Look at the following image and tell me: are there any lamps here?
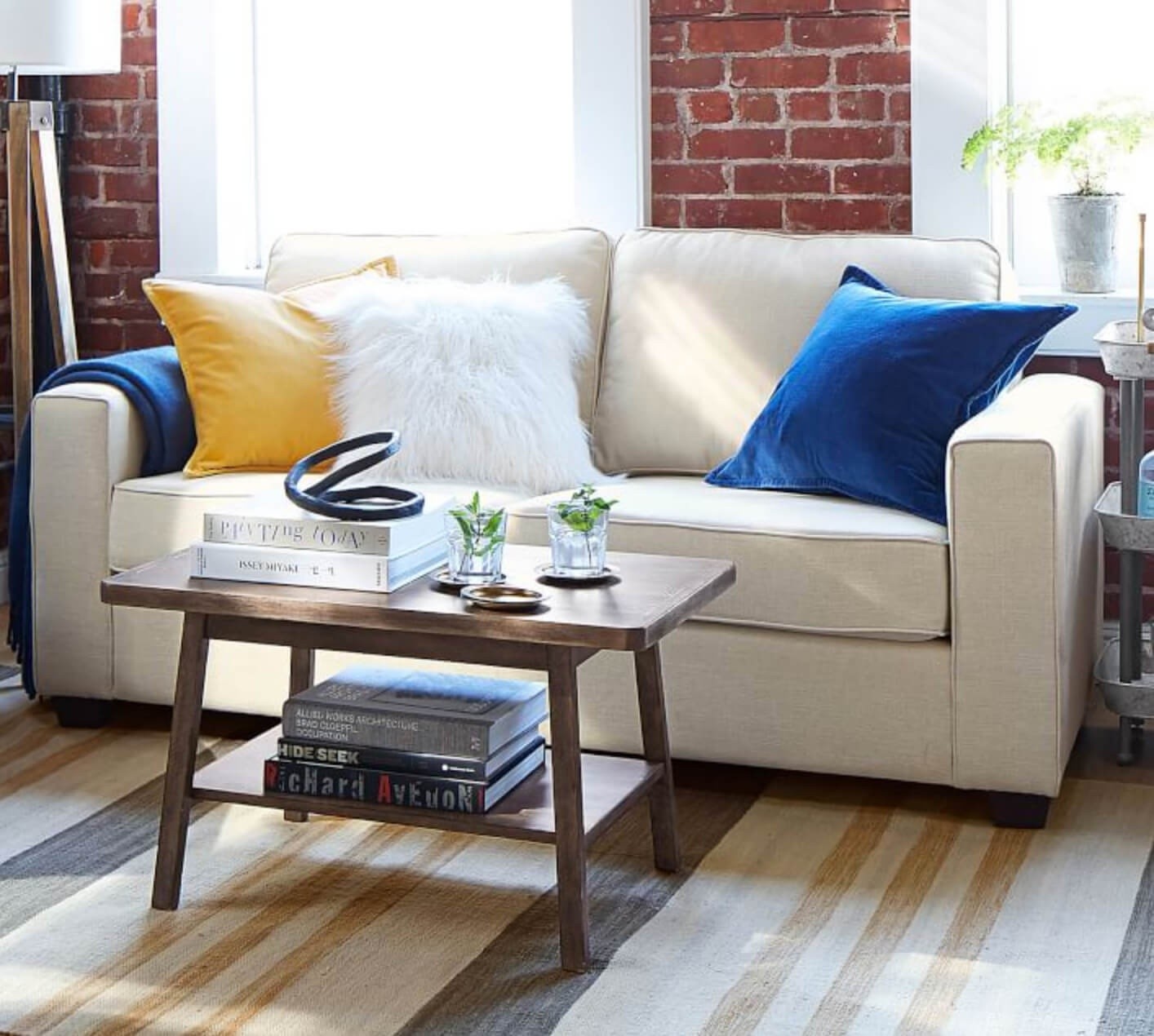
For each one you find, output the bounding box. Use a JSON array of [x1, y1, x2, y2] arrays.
[[0, 0, 121, 465]]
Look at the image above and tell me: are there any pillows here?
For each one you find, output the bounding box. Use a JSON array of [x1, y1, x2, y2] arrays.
[[704, 264, 1078, 525], [138, 257, 397, 479], [346, 272, 607, 490]]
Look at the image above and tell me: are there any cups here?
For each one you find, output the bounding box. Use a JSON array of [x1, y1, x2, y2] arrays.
[[546, 502, 610, 575], [443, 512, 508, 583]]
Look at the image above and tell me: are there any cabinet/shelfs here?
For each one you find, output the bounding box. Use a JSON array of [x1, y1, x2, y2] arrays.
[[1094, 318, 1154, 766]]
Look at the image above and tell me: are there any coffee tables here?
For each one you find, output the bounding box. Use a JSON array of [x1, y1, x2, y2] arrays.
[[102, 536, 742, 975]]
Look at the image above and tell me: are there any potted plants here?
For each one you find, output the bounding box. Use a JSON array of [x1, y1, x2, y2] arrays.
[[537, 477, 622, 579], [961, 105, 1144, 294], [439, 492, 509, 585]]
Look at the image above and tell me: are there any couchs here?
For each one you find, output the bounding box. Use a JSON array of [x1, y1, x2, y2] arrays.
[[23, 226, 1106, 831]]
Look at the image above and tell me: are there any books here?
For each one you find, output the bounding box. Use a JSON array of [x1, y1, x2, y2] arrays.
[[188, 535, 453, 594], [204, 499, 454, 556], [264, 735, 546, 815], [277, 724, 539, 782], [283, 667, 549, 759]]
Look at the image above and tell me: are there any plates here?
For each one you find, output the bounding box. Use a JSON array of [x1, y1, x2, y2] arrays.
[[533, 563, 614, 581], [427, 567, 509, 585], [461, 584, 550, 610]]
